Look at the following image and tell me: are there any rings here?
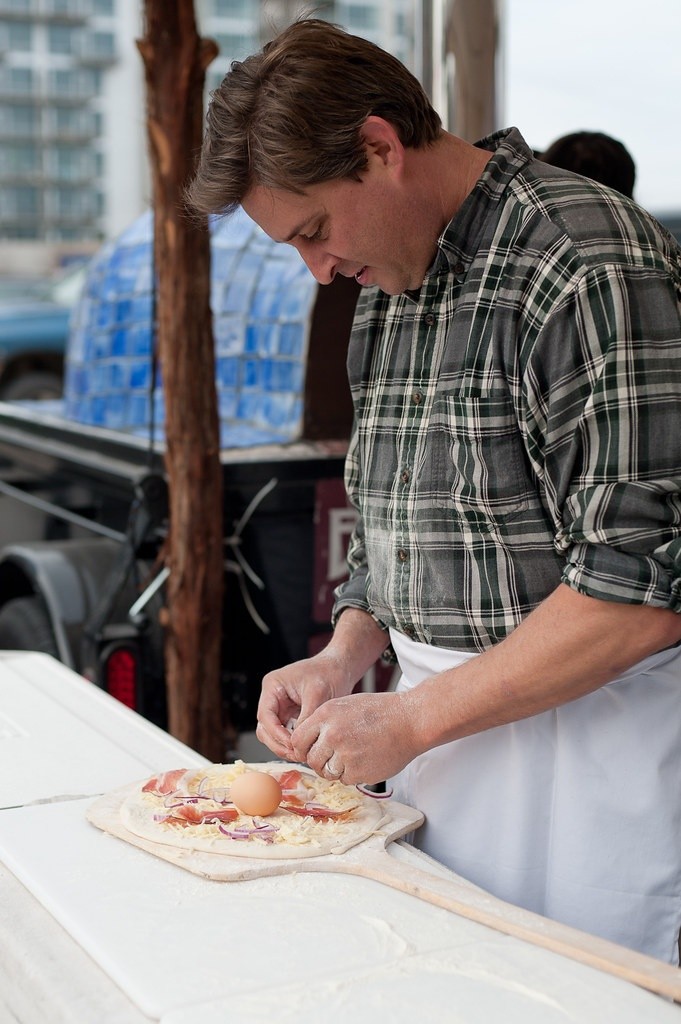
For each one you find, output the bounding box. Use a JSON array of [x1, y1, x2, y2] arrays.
[[325, 762, 344, 775]]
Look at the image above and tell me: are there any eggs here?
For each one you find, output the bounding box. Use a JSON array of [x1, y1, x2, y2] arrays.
[[230, 771, 283, 817]]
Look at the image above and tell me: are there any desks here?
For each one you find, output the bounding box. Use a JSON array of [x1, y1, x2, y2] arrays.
[[1, 398, 351, 672], [0, 647, 681, 1024]]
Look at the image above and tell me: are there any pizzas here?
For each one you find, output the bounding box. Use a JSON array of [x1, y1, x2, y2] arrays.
[[117, 759, 390, 859]]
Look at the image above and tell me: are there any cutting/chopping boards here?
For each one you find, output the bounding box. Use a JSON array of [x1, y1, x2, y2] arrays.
[[89, 763, 681, 1004]]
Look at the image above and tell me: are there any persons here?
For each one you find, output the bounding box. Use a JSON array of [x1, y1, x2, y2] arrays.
[[544, 132, 636, 200], [180, 17, 681, 962]]
